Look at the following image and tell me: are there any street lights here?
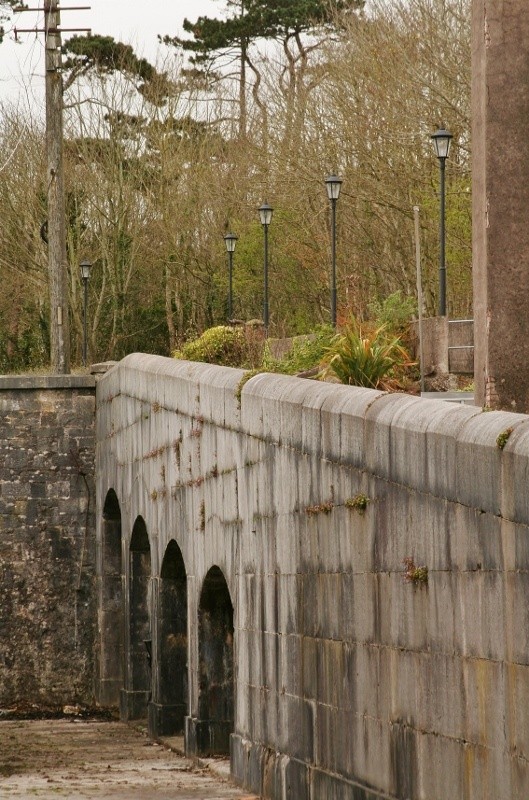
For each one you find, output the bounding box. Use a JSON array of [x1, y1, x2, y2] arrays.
[[325, 175, 344, 327], [257, 204, 274, 323], [223, 233, 239, 318], [429, 128, 455, 318], [80, 260, 92, 360]]
